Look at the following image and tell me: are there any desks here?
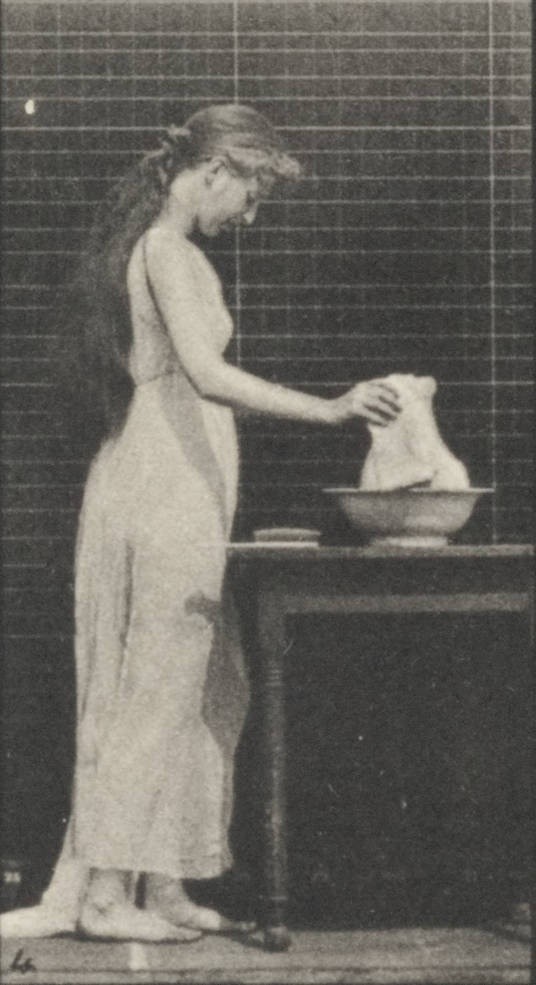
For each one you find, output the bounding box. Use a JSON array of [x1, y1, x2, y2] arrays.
[[228, 541, 535, 955]]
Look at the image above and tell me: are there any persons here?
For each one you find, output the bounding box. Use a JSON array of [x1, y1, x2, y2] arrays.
[[72, 103, 403, 946]]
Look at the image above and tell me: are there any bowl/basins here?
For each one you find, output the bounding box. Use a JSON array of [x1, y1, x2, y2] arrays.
[[320, 487, 496, 546]]
